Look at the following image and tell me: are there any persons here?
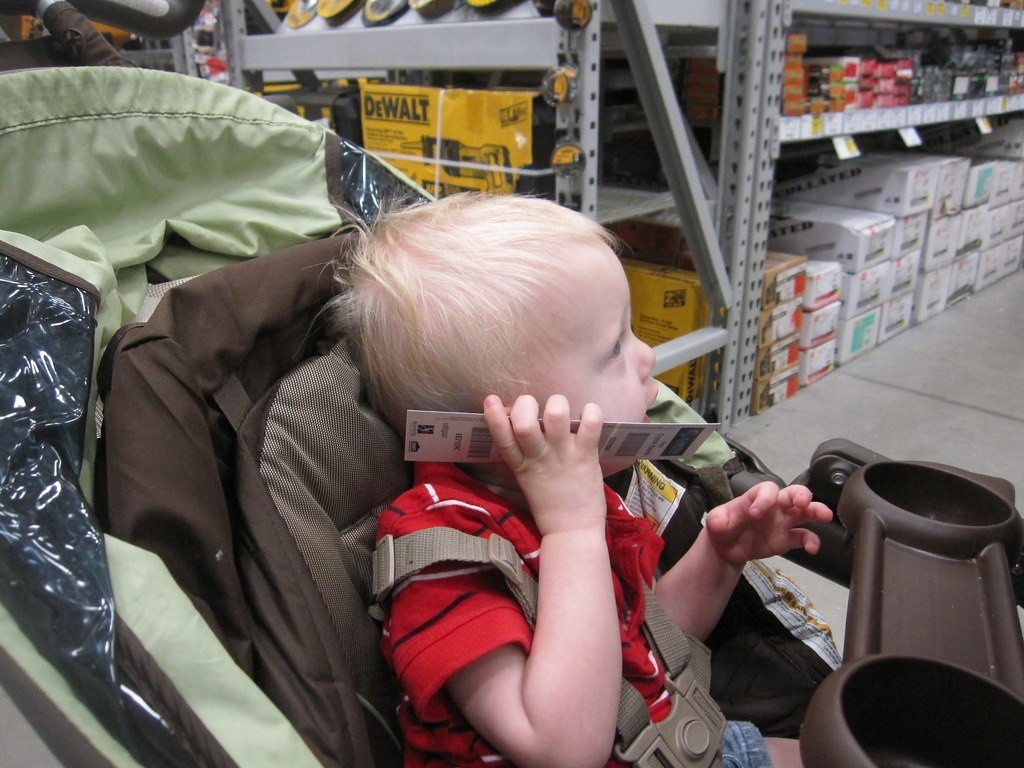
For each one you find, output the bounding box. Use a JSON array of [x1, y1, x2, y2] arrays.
[[337, 189, 834, 768]]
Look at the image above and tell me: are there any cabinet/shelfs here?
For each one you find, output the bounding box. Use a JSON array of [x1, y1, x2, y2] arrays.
[[224, 0, 770, 427], [736, 0, 1024, 419]]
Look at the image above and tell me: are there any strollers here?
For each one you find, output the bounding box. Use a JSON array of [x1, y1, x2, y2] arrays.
[[0, 0, 1024, 768]]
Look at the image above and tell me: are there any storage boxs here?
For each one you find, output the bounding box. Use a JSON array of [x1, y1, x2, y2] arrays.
[[254, 0, 1024, 415]]
